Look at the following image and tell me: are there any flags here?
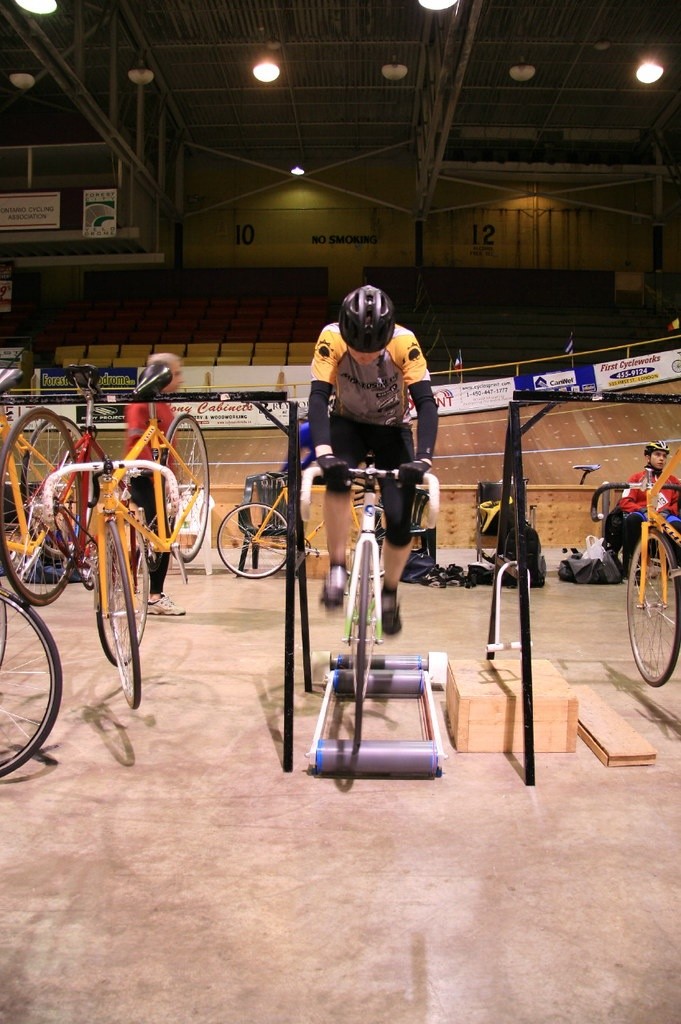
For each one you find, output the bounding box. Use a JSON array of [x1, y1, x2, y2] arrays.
[[564, 334, 575, 354], [667, 318, 680, 332], [454, 353, 460, 369]]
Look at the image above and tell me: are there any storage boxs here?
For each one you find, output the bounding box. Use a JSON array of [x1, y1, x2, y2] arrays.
[[445, 657, 578, 751]]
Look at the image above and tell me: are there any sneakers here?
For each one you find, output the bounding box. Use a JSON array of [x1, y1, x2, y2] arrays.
[[146, 593, 186, 615], [445, 563, 466, 587], [419, 564, 446, 588]]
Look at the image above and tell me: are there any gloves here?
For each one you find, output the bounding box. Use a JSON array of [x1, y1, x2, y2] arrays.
[[317, 454, 349, 484], [659, 510, 671, 518], [398, 459, 431, 485], [640, 507, 648, 518]]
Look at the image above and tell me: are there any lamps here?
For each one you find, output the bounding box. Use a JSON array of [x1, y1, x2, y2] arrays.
[[381, 55, 408, 81], [8, 70, 36, 88], [508, 55, 536, 82], [253, 54, 280, 82], [127, 50, 155, 86]]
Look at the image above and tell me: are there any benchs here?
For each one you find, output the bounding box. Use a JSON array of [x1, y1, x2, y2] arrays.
[[366, 294, 681, 389]]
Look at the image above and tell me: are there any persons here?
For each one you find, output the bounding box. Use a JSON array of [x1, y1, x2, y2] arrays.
[[124, 351, 186, 615], [308, 283, 440, 636], [617, 437, 680, 584]]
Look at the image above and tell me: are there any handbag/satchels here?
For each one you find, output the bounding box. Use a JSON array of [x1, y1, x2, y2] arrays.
[[559, 550, 625, 584], [581, 535, 607, 561], [465, 562, 494, 588], [400, 552, 435, 583]]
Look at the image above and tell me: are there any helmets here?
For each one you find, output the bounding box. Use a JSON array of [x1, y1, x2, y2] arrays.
[[644, 440, 670, 456], [339, 285, 395, 353]]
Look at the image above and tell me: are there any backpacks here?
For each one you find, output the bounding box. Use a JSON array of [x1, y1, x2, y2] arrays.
[[504, 525, 546, 588]]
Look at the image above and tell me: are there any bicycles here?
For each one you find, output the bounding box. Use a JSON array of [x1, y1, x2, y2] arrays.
[[216, 471, 387, 580], [590, 482, 681, 687], [299, 467, 439, 754], [0, 582, 64, 777], [42, 365, 211, 709], [0, 363, 149, 666]]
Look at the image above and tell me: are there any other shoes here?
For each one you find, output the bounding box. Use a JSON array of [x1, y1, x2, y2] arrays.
[[323, 564, 346, 607], [381, 589, 401, 635]]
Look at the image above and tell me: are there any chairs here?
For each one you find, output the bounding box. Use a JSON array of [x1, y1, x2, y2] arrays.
[[476, 481, 534, 558], [171, 489, 214, 576], [234, 471, 287, 575], [0, 294, 329, 381], [408, 488, 430, 557]]
